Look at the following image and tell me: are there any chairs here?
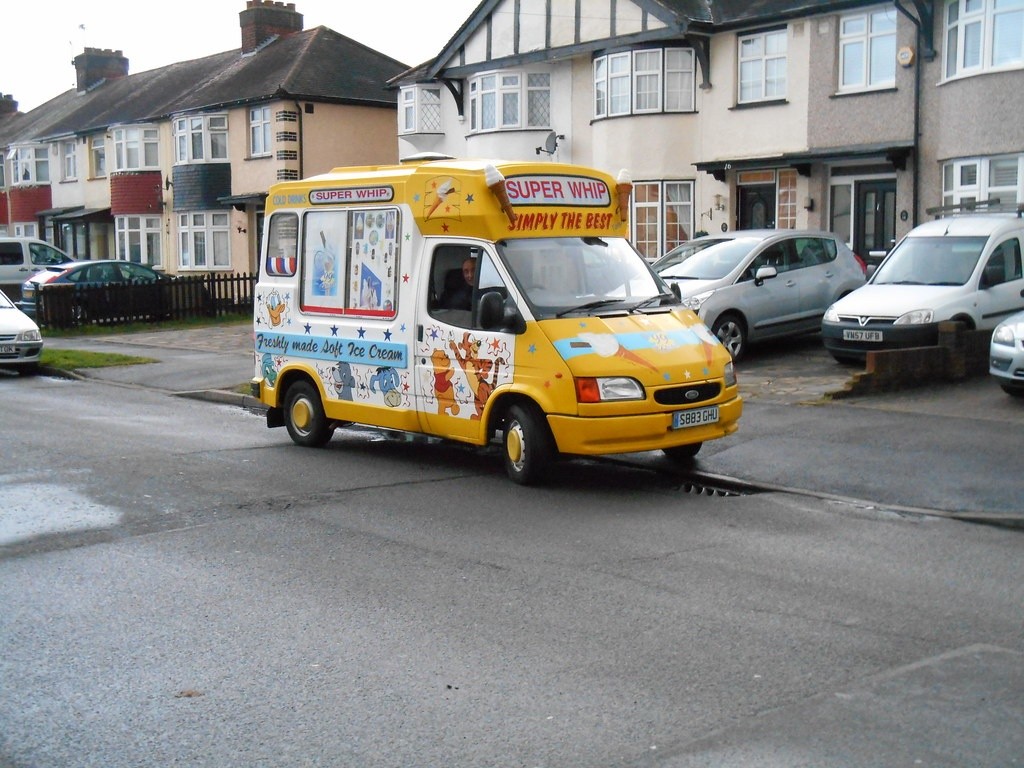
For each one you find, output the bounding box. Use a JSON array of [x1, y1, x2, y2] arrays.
[[760, 245, 783, 267], [442, 268, 473, 308], [90, 269, 104, 283]]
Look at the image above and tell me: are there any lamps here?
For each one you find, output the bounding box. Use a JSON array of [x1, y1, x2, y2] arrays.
[[236, 221, 247, 233], [803, 197, 814, 212], [713, 194, 724, 211]]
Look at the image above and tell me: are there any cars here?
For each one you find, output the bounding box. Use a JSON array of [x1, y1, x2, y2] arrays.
[[0, 289, 44, 376], [14, 258, 208, 328], [603, 227, 868, 366], [988, 289, 1024, 396], [820, 213, 1024, 384]]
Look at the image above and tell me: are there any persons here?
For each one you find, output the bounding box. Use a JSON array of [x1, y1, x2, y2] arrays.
[[444, 257, 477, 311]]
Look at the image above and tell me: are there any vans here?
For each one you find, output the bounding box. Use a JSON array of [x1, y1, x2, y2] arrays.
[[0, 238, 106, 309]]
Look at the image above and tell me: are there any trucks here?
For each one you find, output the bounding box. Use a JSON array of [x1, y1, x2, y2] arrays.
[[249, 158, 744, 487]]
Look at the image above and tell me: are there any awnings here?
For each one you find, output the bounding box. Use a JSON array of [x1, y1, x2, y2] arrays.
[[785, 142, 913, 176], [35, 205, 84, 246], [217, 192, 269, 212], [692, 156, 811, 182], [49, 208, 114, 260]]
